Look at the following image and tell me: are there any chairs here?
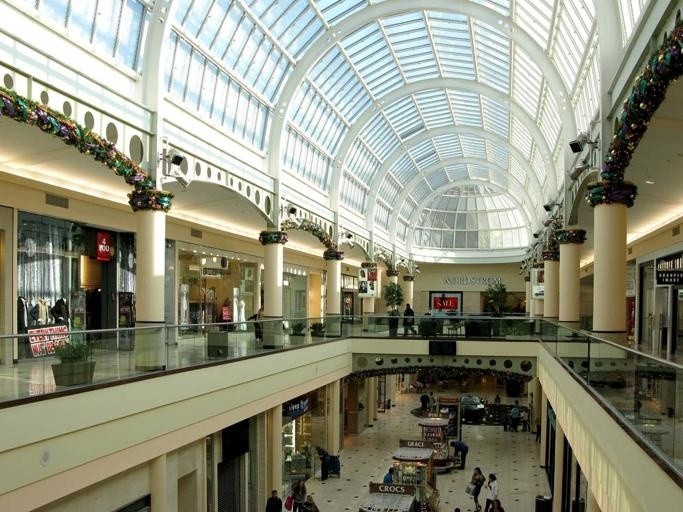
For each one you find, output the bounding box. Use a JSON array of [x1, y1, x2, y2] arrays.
[[421, 321, 436, 340]]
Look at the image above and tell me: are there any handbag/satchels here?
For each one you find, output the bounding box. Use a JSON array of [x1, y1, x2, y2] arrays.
[[284, 495, 294, 511], [464, 481, 476, 496]]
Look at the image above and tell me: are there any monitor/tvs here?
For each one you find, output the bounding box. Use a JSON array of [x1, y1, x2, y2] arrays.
[[429, 340, 456, 356], [220, 418, 249, 463]]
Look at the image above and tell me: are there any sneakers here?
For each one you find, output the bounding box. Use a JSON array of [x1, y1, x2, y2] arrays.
[[473, 505, 481, 512]]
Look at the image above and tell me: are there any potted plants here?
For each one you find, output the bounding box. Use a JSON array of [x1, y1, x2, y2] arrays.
[[384, 282, 404, 336], [289, 322, 305, 346], [488, 283, 507, 336], [52, 342, 97, 386]]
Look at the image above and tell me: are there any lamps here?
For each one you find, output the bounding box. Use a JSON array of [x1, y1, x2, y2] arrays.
[[342, 231, 352, 238], [570, 132, 595, 153], [283, 203, 296, 214], [534, 229, 543, 238], [544, 199, 561, 211], [160, 148, 183, 166]]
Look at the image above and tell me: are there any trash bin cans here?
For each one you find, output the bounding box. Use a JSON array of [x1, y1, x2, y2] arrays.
[[535, 495, 553, 512], [572, 497, 585, 512], [207, 330, 228, 361]]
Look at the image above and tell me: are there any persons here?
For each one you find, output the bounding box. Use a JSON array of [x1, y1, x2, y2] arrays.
[[289, 479, 306, 512], [264, 490, 282, 512], [453, 507, 460, 512], [400, 303, 417, 337], [358, 281, 367, 293], [49, 297, 71, 325], [427, 391, 435, 412], [469, 467, 486, 512], [419, 391, 429, 412], [246, 308, 263, 340], [484, 473, 498, 512], [314, 445, 330, 482], [30, 298, 53, 325], [414, 378, 424, 394], [534, 415, 540, 444], [488, 499, 505, 512], [493, 393, 500, 404], [502, 406, 511, 432], [449, 441, 468, 470], [382, 466, 394, 485], [17, 295, 28, 331], [509, 404, 519, 433], [519, 406, 527, 432], [218, 297, 231, 331], [300, 494, 319, 512]]
